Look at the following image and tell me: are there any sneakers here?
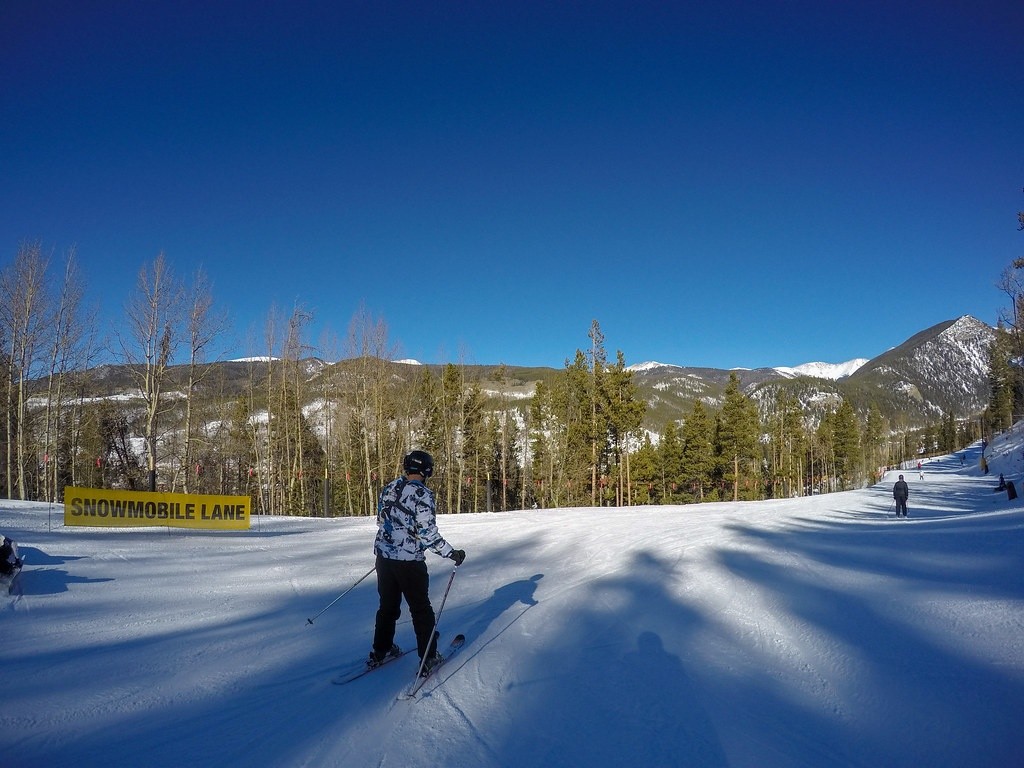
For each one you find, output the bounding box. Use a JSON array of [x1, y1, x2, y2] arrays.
[[370, 642, 399, 663], [419, 649, 443, 676]]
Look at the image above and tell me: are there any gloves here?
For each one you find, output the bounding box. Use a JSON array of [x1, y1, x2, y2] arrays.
[[14, 558, 23, 569], [447, 549, 466, 566]]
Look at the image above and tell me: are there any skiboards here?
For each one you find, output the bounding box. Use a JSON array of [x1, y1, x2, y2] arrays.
[[331, 630, 466, 700]]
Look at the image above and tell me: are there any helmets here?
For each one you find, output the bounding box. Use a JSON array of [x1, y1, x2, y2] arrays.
[[403, 451, 434, 478]]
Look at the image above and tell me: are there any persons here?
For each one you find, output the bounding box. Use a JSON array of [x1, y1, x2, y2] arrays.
[[918, 462, 921, 469], [369, 450, 466, 672], [893, 475, 908, 519], [0, 535, 21, 577], [919, 470, 924, 480]]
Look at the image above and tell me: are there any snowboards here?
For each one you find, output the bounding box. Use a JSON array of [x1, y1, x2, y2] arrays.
[[7, 555, 26, 597]]
